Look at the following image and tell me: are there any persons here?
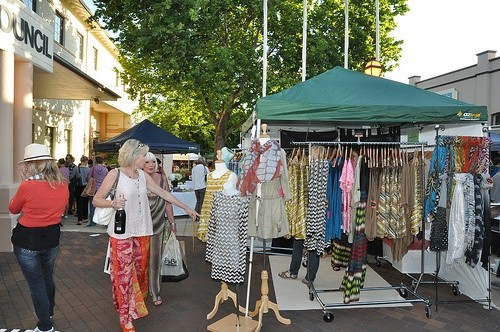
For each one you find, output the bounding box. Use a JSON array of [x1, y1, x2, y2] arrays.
[[279, 145, 326, 283], [196, 161, 238, 241], [154, 159, 209, 223], [205, 181, 249, 283], [91, 139, 200, 332], [489, 156, 500, 205], [56, 154, 112, 228], [141, 152, 177, 305], [9, 143, 70, 332]]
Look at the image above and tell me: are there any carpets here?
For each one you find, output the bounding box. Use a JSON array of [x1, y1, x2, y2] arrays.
[[269, 255, 413, 311]]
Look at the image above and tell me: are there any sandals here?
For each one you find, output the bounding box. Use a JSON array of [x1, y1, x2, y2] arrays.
[[278, 271, 297, 278], [302, 277, 313, 286], [152, 295, 163, 306], [331, 262, 340, 270], [120, 321, 136, 331]]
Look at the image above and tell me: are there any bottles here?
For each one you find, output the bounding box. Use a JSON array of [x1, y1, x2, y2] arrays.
[[114, 194, 126, 234]]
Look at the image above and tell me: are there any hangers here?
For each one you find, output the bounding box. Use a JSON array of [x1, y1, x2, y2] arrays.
[[290, 142, 420, 168]]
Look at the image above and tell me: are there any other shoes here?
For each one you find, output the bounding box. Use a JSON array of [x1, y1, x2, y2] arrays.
[[61, 213, 68, 218], [77, 218, 88, 224]]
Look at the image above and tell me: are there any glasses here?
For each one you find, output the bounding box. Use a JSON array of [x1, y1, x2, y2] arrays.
[[136, 140, 144, 150]]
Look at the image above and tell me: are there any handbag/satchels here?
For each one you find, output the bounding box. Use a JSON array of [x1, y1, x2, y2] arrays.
[[92, 167, 121, 225], [80, 166, 96, 197], [160, 229, 190, 282], [104, 240, 111, 274], [69, 165, 83, 186], [204, 165, 207, 182]]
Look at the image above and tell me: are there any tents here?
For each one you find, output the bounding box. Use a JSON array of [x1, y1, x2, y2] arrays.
[[242, 65, 491, 316], [94, 118, 201, 191]]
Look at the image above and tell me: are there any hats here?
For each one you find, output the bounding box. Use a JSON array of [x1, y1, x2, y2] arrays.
[[16, 143, 57, 164]]
